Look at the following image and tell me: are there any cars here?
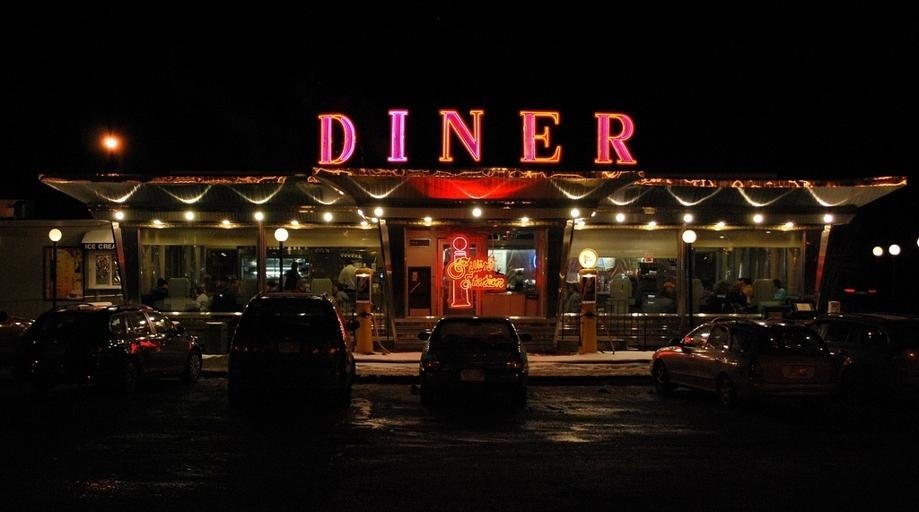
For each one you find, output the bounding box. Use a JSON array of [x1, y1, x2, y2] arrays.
[[0, 310, 35, 352], [837, 277, 880, 301]]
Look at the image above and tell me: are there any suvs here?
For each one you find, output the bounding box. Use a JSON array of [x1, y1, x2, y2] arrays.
[[806, 312, 919, 385], [15, 300, 204, 393], [649, 315, 833, 406], [416, 315, 530, 413], [228, 290, 355, 407]]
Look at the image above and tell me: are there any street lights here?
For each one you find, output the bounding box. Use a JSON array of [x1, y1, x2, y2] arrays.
[[48, 228, 62, 308], [679, 230, 699, 329], [273, 227, 291, 296]]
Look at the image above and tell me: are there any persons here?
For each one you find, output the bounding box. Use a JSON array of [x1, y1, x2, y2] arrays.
[[279, 257, 360, 307], [146, 268, 242, 311], [570, 258, 788, 316]]
